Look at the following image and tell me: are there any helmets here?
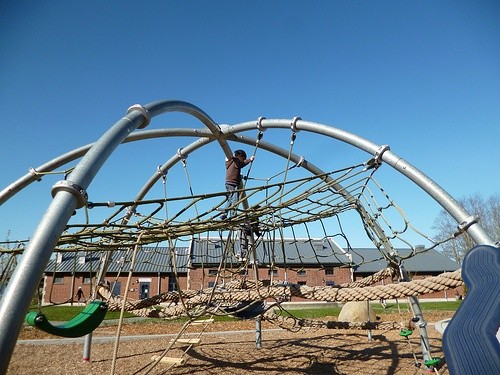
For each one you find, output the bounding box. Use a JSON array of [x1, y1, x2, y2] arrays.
[[235, 150, 246, 160]]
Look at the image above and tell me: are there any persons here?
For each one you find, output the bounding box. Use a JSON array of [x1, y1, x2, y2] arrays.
[[75, 286, 86, 303], [219, 148, 254, 219]]
